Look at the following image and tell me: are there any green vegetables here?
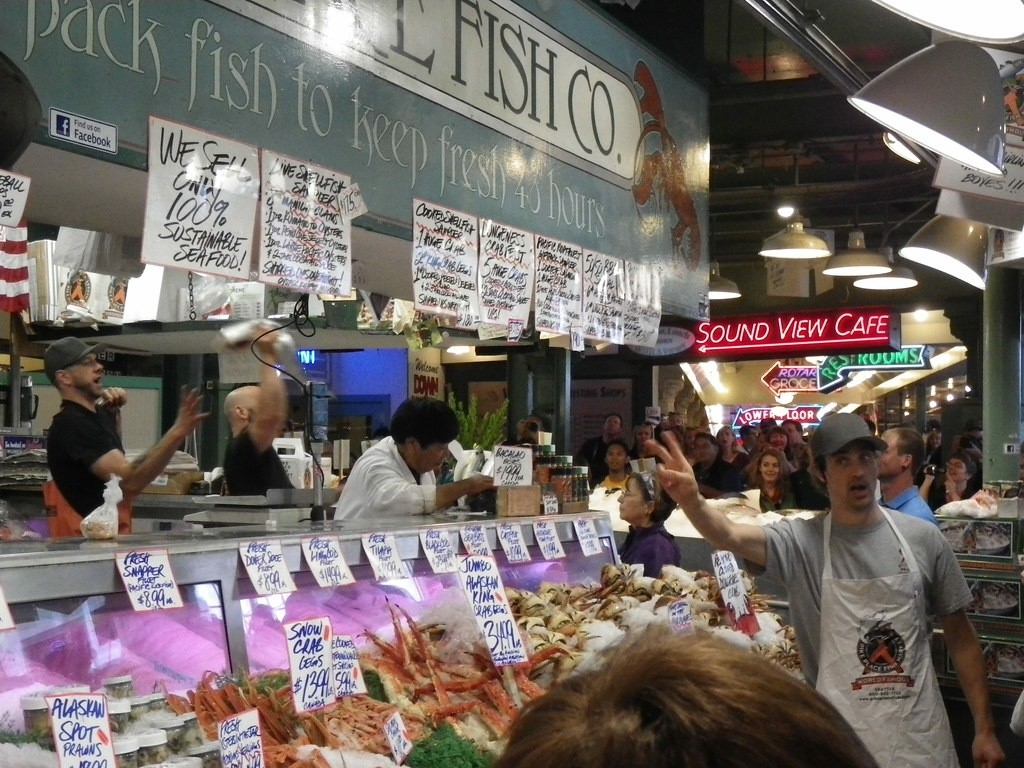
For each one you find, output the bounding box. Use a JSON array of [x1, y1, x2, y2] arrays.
[[212, 668, 499, 768]]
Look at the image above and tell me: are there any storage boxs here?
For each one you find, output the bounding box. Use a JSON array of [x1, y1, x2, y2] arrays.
[[480, 485, 541, 517]]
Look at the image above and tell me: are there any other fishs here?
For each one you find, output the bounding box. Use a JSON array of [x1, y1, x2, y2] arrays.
[[157, 669, 344, 768], [357, 596, 574, 752], [505, 564, 803, 688]]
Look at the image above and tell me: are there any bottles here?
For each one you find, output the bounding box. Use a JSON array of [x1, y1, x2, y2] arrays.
[[532, 443, 591, 503]]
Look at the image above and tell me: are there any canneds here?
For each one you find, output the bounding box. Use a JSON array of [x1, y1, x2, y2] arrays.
[[523, 443, 589, 504]]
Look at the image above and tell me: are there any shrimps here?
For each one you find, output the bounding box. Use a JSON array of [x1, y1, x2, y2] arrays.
[[327, 695, 428, 754]]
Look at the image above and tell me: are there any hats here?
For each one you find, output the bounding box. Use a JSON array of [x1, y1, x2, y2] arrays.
[[44, 337, 101, 381], [810, 413, 888, 461], [962, 419, 983, 430]]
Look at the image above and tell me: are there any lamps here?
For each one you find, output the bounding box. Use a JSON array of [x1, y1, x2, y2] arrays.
[[707, 0, 1024, 300]]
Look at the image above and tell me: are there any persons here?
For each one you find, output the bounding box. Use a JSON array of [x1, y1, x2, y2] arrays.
[[863, 418, 878, 438], [593, 440, 630, 508], [576, 412, 627, 485], [497, 621, 882, 768], [654, 412, 810, 500], [221, 327, 296, 497], [646, 412, 1008, 768], [630, 422, 663, 465], [614, 471, 682, 579], [740, 447, 799, 513], [1019, 440, 1024, 484], [918, 418, 983, 515], [44, 336, 212, 536], [875, 427, 939, 528], [518, 407, 553, 446], [333, 395, 493, 522]]
